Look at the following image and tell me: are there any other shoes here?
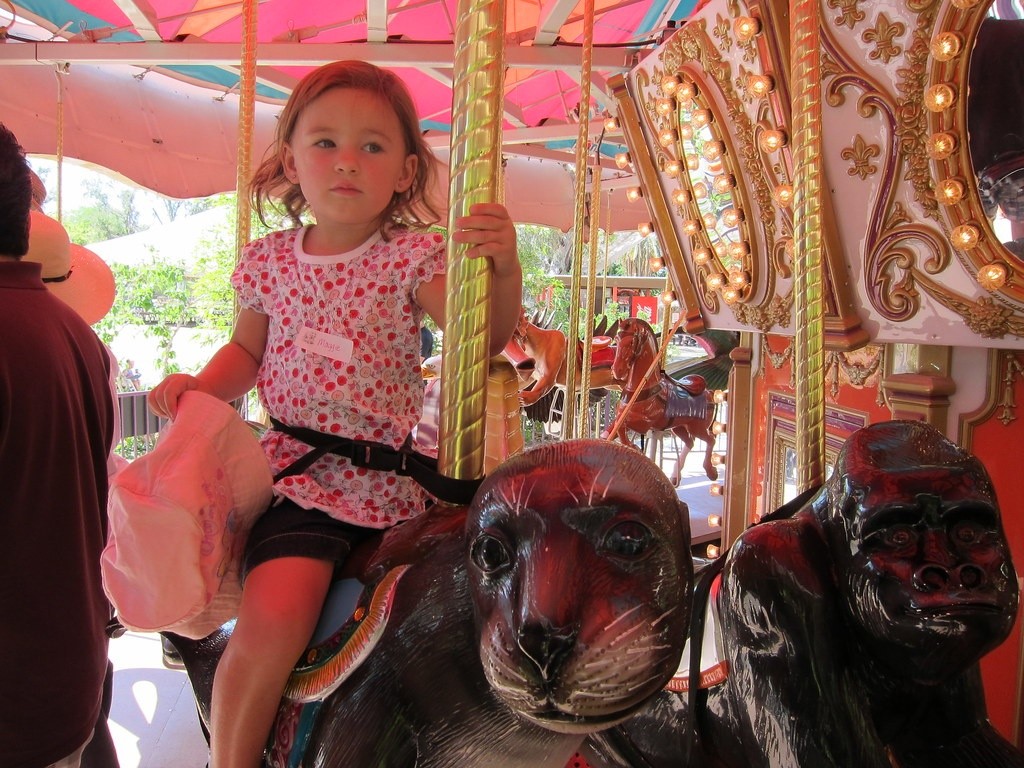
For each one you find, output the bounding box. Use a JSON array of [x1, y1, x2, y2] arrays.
[[105, 616, 128, 638], [162, 638, 186, 670]]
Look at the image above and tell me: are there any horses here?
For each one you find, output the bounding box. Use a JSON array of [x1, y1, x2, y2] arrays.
[[514, 308, 718, 489]]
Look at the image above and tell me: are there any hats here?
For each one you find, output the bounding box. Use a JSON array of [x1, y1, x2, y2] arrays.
[[21, 209, 116, 327], [100, 390, 273, 641], [28, 166, 47, 215]]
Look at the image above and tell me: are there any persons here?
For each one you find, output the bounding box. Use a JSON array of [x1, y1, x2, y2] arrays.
[[152, 61, 522, 768], [0, 123, 121, 768]]
